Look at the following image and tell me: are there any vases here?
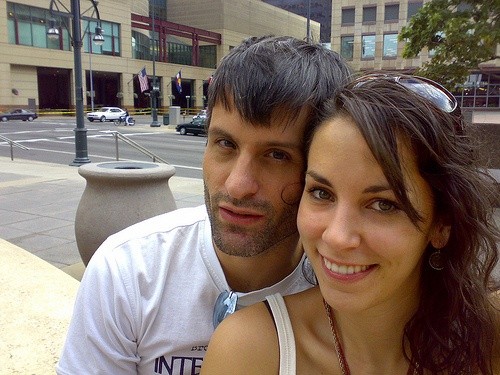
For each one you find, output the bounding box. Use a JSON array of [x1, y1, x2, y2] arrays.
[[74, 160, 178, 269]]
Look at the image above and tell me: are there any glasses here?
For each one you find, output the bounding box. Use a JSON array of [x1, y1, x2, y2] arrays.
[[213, 289, 238, 330], [350, 73, 464, 139]]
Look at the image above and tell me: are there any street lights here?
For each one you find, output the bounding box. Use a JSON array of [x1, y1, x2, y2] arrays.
[[46, 0, 105, 167]]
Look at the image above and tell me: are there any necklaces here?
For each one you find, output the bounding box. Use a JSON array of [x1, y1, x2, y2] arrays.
[[323, 297, 420, 375]]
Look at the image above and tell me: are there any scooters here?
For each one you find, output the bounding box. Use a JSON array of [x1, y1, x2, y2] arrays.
[[114, 116, 135, 126]]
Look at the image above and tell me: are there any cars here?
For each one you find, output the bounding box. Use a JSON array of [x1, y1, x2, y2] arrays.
[[174, 110, 207, 135], [86, 106, 127, 122], [0, 108, 38, 122]]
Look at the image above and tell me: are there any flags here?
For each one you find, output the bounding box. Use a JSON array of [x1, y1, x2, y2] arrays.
[[174, 71, 182, 92], [138, 67, 149, 92]]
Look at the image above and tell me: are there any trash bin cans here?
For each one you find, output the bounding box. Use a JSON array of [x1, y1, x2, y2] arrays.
[[163, 116, 169, 125], [169, 106, 180, 125]]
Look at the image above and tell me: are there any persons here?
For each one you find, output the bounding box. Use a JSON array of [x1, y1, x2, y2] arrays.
[[200, 70, 500, 375], [122, 108, 129, 126], [56, 32, 352, 375]]
